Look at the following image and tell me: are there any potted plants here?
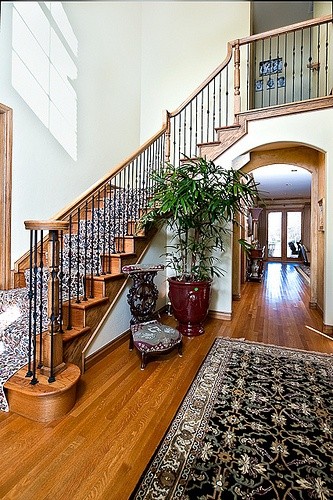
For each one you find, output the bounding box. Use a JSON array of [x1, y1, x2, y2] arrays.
[[147, 157, 263, 337]]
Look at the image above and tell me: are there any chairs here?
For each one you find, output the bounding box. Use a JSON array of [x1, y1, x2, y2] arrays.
[[296, 241, 310, 266], [121, 263, 184, 371]]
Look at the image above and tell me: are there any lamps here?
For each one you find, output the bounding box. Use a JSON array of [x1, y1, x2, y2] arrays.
[[249, 207, 263, 246]]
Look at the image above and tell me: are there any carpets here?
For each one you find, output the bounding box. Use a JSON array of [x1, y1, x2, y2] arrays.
[[126, 335, 333, 500], [294, 265, 310, 284]]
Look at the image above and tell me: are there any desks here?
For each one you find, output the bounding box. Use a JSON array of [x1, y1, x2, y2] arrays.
[[247, 245, 266, 282]]
